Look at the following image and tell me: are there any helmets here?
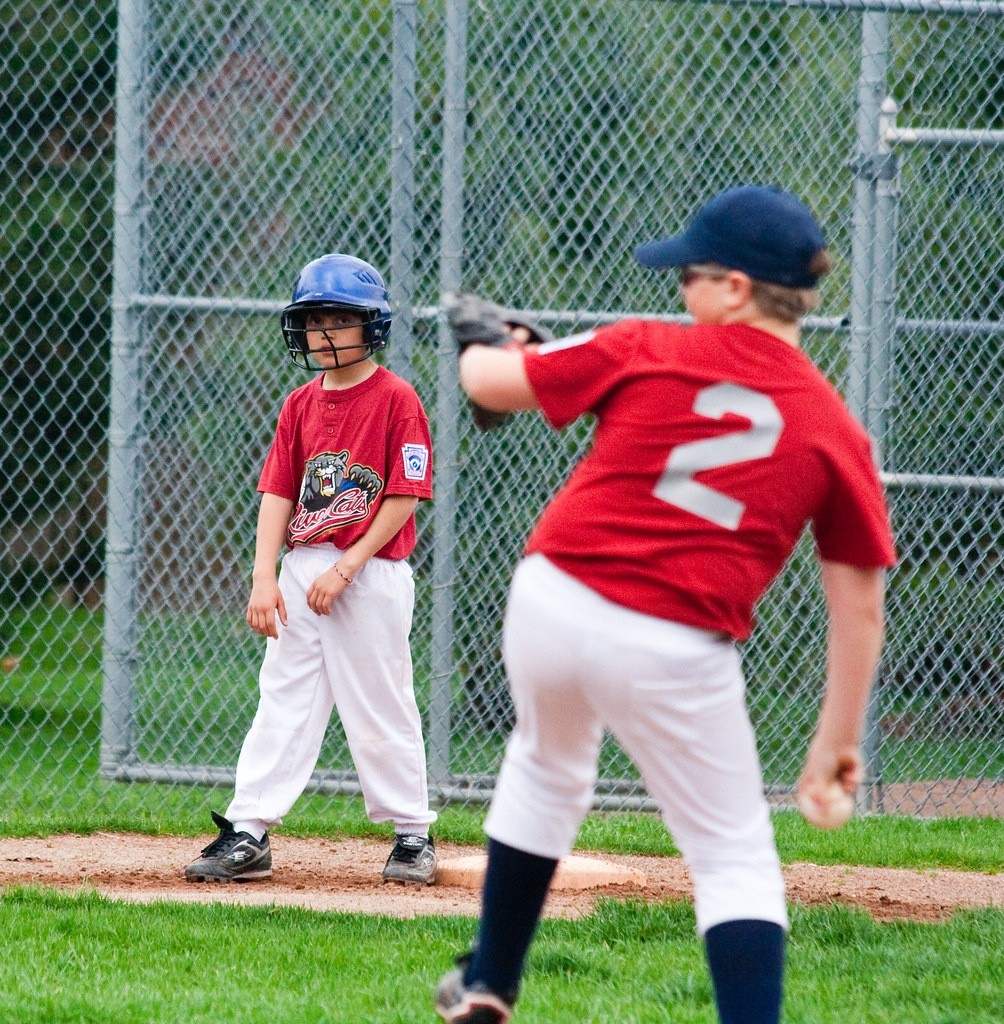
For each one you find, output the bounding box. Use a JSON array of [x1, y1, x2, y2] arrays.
[[281, 254, 391, 371]]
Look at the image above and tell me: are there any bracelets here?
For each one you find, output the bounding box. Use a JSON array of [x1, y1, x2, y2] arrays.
[[334, 563, 353, 583]]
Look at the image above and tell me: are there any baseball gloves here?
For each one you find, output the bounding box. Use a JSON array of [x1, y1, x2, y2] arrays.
[[441, 291, 557, 435]]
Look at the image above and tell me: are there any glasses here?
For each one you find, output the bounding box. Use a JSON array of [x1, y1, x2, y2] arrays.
[[677, 264, 725, 284]]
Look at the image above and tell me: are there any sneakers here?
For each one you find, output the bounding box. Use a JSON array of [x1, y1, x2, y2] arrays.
[[190, 812, 274, 877], [436, 968, 513, 1024], [386, 833, 438, 886]]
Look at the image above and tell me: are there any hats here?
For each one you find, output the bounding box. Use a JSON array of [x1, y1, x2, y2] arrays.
[[636, 184, 827, 288]]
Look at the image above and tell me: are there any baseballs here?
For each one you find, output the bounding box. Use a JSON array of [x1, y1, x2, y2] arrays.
[[797, 775, 857, 831]]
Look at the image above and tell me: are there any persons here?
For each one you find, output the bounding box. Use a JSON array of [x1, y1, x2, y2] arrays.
[[434, 186, 897, 1024], [184, 253, 437, 883]]
